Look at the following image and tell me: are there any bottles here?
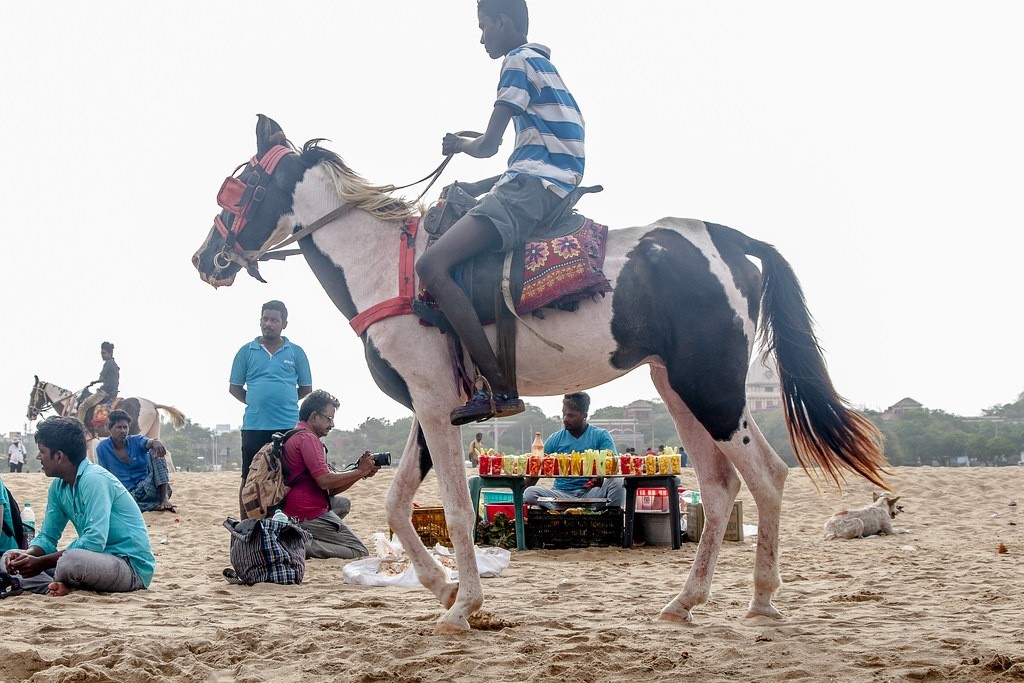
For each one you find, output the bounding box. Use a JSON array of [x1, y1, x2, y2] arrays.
[[272, 509, 288, 522], [20, 503, 36, 548], [531, 432, 545, 459]]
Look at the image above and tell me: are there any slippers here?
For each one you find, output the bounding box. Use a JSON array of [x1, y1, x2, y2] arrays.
[[451, 390, 525, 426]]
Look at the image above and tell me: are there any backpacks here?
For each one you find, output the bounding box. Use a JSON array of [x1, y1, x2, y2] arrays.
[[242, 428, 328, 519], [2, 485, 28, 551]]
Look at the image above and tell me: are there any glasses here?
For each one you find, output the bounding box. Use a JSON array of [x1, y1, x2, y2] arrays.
[[314, 409, 333, 422]]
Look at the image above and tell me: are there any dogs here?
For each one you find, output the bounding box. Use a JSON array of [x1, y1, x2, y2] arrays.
[[824, 491, 900, 540]]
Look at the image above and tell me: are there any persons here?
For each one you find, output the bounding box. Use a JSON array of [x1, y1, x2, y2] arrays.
[[625, 445, 687, 467], [8, 436, 28, 473], [469, 433, 484, 468], [228, 299, 313, 521], [96, 408, 178, 513], [78, 342, 120, 441], [0, 415, 156, 597], [523, 392, 627, 511], [412, 0, 586, 428], [280, 389, 381, 560]]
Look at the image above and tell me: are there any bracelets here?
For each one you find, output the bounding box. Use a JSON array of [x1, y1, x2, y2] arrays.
[[24, 459, 27, 460]]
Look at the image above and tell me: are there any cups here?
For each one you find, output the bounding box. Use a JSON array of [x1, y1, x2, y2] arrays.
[[478, 453, 682, 476]]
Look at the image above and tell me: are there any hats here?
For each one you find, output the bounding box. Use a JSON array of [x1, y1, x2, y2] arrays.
[[11, 437, 19, 443]]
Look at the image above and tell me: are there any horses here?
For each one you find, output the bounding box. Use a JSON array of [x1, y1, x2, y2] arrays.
[[27, 375, 187, 464], [191, 114, 895, 639]]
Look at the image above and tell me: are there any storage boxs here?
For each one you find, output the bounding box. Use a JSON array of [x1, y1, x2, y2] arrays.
[[525, 509, 622, 549], [481, 490, 527, 522], [388, 506, 452, 547], [687, 498, 743, 542]]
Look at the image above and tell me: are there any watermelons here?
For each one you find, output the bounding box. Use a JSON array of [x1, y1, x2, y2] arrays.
[[620, 456, 642, 476], [480, 452, 502, 475]]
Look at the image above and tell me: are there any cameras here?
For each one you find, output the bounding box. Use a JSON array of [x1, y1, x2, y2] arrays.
[[357, 451, 392, 468]]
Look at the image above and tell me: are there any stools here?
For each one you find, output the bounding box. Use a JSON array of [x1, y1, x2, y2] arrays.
[[624, 477, 682, 548], [468, 475, 525, 551]]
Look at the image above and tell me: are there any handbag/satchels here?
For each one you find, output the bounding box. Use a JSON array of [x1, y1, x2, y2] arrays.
[[222, 517, 306, 587], [469, 453, 473, 462]]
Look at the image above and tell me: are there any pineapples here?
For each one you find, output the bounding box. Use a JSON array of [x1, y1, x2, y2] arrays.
[[477, 511, 516, 550], [645, 454, 682, 475]]
